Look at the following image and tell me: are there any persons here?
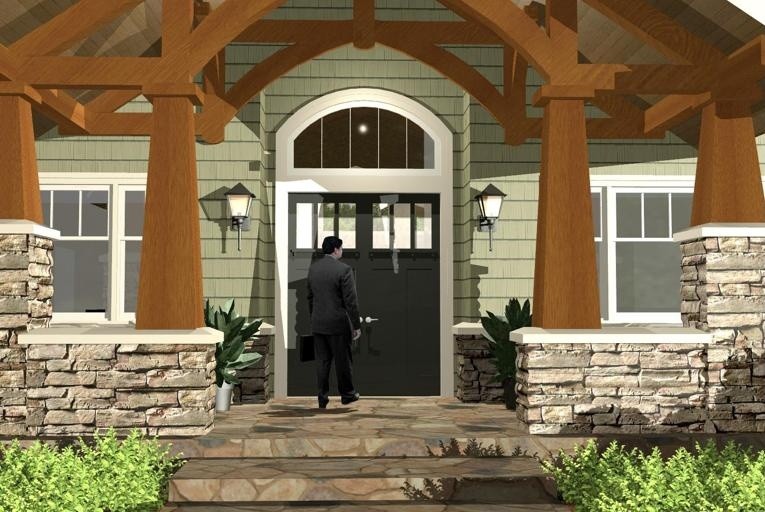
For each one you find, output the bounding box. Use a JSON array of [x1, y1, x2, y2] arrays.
[[303, 236, 365, 411]]
